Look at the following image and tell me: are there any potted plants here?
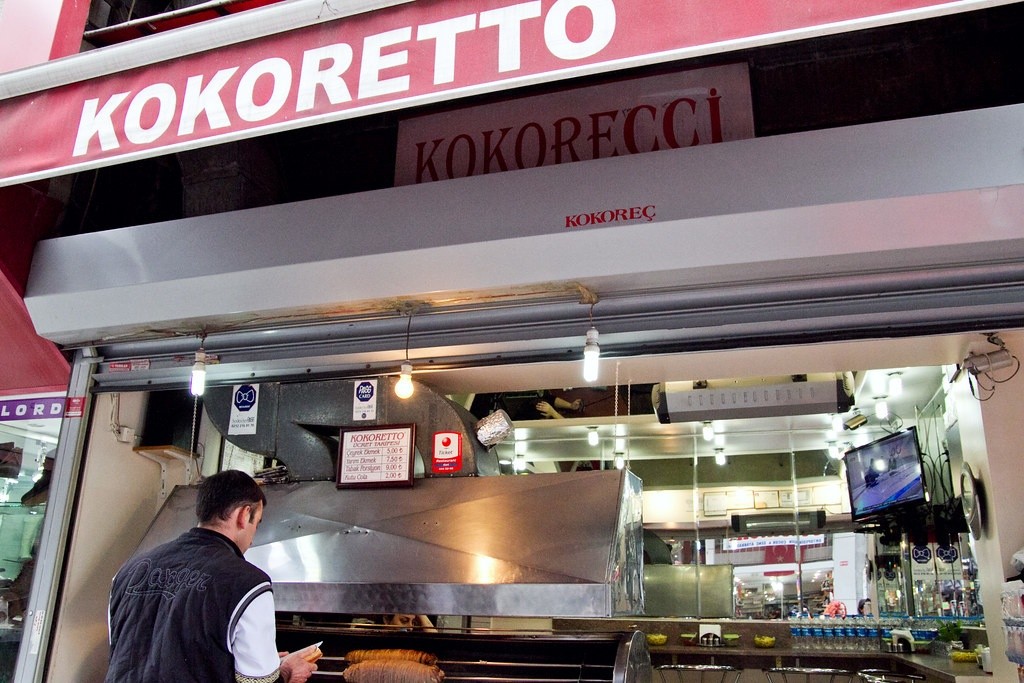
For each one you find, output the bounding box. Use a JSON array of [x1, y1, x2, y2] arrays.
[[936, 620, 964, 656]]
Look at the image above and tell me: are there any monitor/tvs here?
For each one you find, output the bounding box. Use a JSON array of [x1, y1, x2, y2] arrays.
[[843, 425, 925, 520]]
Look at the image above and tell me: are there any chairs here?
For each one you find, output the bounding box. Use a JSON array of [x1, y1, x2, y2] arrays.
[[654, 665, 741, 683], [856, 669, 926, 683], [762, 667, 854, 683]]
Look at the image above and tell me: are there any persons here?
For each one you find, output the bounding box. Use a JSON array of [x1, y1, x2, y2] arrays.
[[857, 597, 871, 614], [382, 613, 438, 632], [103, 470, 318, 683], [820, 598, 846, 618], [865, 454, 897, 488]]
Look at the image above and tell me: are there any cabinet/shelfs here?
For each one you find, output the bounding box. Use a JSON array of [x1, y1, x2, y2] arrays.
[[0, 417, 63, 683]]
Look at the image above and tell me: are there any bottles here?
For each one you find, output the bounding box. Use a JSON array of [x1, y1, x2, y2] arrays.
[[801, 613, 812, 648], [844, 614, 857, 650], [789, 613, 802, 650], [867, 613, 983, 649], [823, 613, 835, 649], [811, 613, 823, 649], [856, 615, 867, 650], [834, 614, 847, 650]]
